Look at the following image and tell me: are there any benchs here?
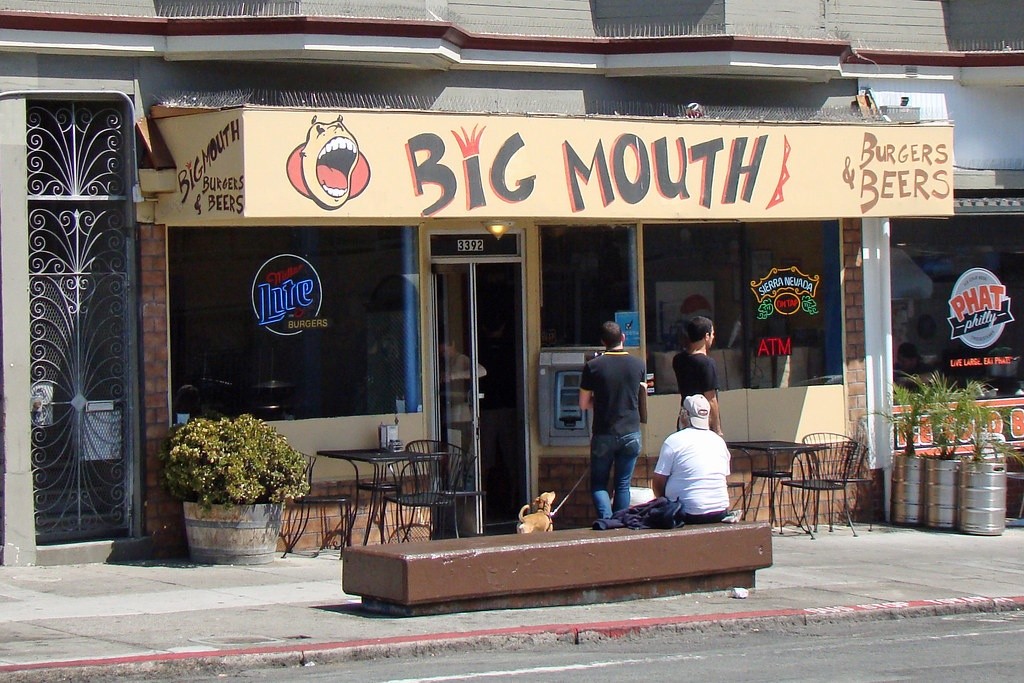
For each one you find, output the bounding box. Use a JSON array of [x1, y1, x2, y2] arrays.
[[343, 522, 772, 617]]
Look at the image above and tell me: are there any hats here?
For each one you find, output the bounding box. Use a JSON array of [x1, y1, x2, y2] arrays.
[[683, 394, 710, 430]]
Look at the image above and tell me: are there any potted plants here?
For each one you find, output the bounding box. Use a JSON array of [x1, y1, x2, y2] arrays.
[[155, 413, 310, 566], [861, 370, 1024, 536]]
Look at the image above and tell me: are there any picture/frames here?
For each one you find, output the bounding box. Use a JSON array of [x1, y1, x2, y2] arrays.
[[733, 249, 773, 302]]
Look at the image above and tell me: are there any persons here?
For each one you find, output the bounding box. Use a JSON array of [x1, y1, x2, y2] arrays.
[[435, 336, 491, 501], [653, 393, 731, 523], [579, 321, 646, 518], [895, 342, 932, 387], [672, 315, 723, 437]]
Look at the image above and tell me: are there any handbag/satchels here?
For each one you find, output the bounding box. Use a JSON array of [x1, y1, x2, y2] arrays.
[[440, 356, 472, 408], [721, 510, 744, 523]]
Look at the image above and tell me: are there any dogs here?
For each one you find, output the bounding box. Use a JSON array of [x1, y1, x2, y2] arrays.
[[516, 491, 556, 534]]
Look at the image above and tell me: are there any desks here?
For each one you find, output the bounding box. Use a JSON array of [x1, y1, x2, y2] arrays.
[[317, 448, 442, 546], [727, 440, 829, 534]]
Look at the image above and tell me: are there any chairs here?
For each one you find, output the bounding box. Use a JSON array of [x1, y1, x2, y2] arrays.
[[726, 433, 873, 539], [281, 450, 353, 560], [381, 439, 477, 544]]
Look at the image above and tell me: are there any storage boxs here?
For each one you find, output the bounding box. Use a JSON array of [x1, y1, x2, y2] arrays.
[[64, 412, 122, 460]]
